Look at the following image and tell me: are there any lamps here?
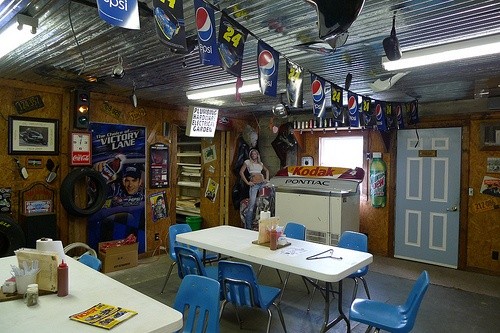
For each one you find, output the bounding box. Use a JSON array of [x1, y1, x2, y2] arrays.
[[382, 12, 403, 61], [382, 35, 500, 71], [186, 80, 263, 99], [130, 80, 138, 107], [113, 57, 125, 79]]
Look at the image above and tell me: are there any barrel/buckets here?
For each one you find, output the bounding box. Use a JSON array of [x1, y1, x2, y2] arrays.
[[186, 216, 202, 231]]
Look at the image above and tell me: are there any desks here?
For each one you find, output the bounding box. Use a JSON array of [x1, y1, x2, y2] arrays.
[[0, 248, 184, 333], [176, 224, 373, 333]]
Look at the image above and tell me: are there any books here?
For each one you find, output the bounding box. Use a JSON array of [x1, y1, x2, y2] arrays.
[[69, 303, 138, 330]]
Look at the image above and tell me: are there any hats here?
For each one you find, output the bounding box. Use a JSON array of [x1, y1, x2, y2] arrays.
[[122, 166, 141, 179]]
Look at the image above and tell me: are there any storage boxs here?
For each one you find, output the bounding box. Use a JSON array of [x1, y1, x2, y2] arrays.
[[98, 239, 138, 273]]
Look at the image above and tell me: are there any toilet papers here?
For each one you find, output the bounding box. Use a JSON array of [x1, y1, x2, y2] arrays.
[[260, 211, 270, 220], [35, 237, 66, 267]]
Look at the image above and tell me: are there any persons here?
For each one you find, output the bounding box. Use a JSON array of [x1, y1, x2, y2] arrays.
[[93, 161, 144, 208], [155, 196, 164, 206], [240, 149, 270, 228]]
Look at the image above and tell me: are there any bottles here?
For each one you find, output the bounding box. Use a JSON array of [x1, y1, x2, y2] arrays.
[[270, 229, 277, 250], [369, 151, 386, 209], [100, 154, 123, 181], [56, 259, 68, 297], [23, 283, 38, 306], [292, 116, 339, 129]]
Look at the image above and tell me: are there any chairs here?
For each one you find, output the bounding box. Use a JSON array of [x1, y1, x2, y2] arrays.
[[160, 223, 431, 333], [79, 255, 102, 271]]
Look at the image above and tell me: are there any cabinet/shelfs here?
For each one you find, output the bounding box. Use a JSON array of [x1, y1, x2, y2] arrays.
[[177, 140, 201, 217]]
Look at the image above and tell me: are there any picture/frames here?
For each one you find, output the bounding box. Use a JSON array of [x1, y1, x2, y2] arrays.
[[8, 115, 59, 156], [25, 157, 44, 169]]
